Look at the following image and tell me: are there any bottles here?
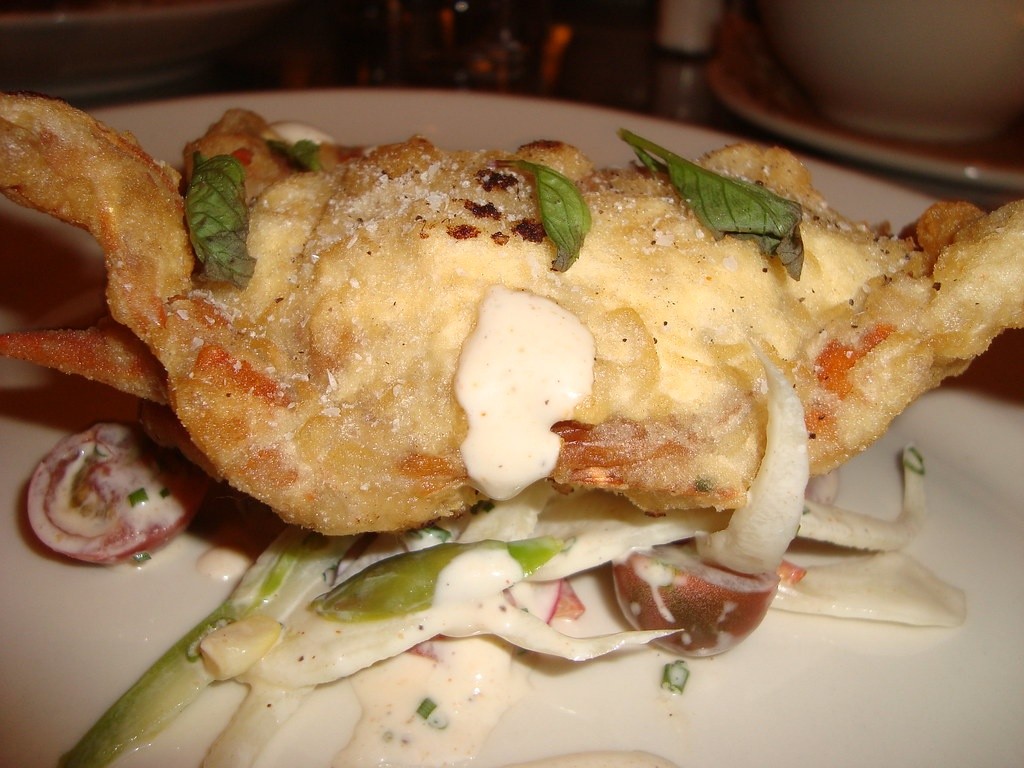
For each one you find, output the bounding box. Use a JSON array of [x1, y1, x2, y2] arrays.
[[654, 0, 725, 60]]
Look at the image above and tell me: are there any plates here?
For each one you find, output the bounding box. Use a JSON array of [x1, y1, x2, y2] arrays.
[[708, 51, 1024, 187], [1, 89, 1024, 768]]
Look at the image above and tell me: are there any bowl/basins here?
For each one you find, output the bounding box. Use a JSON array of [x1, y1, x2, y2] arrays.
[[759, 0, 1024, 146], [0, 0, 291, 98]]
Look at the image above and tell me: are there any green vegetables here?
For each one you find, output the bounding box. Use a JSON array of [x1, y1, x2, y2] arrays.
[[185, 151, 255, 289], [518, 161, 592, 272], [619, 128, 805, 278], [267, 138, 322, 172]]
